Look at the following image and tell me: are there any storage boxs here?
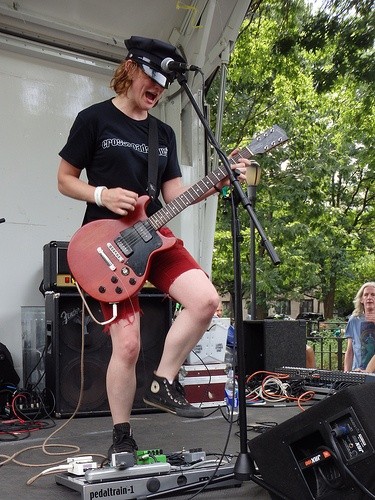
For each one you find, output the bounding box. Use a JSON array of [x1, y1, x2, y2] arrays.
[[186, 318, 231, 364], [180, 364, 215, 408]]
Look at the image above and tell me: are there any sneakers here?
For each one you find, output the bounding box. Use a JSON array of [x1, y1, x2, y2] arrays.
[[143, 370, 205, 418], [108, 421, 138, 463]]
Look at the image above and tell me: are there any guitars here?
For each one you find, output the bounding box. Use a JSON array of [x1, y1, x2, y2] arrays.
[[67, 123, 291, 304]]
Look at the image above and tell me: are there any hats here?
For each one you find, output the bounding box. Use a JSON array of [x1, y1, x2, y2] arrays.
[[124, 36, 187, 89]]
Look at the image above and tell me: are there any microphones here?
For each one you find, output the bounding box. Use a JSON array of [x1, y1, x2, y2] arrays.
[[160, 57, 199, 73]]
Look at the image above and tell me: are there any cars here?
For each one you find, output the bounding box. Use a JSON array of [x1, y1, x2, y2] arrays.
[[294, 312, 325, 322], [264, 313, 295, 320]]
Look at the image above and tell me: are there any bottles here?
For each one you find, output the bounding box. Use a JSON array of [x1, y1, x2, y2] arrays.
[[224, 370, 239, 416]]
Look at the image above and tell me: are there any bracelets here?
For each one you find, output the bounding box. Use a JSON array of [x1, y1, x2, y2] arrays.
[[94, 186, 107, 206], [214, 184, 222, 192]]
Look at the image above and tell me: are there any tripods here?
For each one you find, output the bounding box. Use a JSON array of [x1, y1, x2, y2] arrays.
[[136, 71, 290, 500]]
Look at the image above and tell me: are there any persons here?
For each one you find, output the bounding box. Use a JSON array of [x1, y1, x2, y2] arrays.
[[58, 35, 251, 455], [344, 282, 375, 374], [275, 314, 315, 368], [213, 297, 237, 349], [229, 318, 235, 326]]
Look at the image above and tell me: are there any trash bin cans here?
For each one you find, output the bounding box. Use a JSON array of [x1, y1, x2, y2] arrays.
[[301, 312, 322, 337], [266, 315, 288, 320]]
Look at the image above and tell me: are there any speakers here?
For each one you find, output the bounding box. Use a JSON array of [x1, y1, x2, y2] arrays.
[[242, 318, 307, 384], [250, 381, 375, 500], [43, 291, 176, 418]]
